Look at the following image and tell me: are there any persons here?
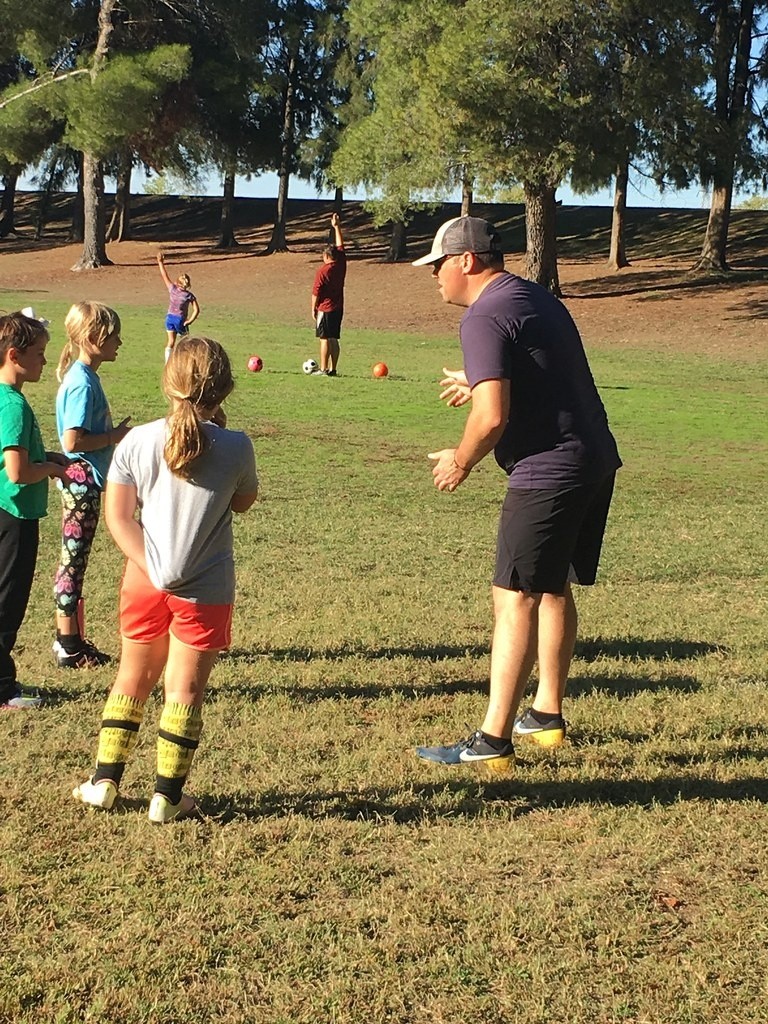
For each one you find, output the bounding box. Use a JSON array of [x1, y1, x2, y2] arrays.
[[72, 337, 259, 824], [157, 252, 199, 364], [53, 301, 136, 669], [311, 213, 347, 377], [0, 311, 71, 708], [412, 216, 623, 769]]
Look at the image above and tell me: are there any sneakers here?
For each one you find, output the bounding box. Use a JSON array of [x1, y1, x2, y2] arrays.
[[512, 714, 566, 746], [311, 370, 336, 376], [149, 792, 196, 823], [71, 775, 118, 809], [416, 730, 516, 770], [52, 639, 112, 668], [4, 690, 45, 708]]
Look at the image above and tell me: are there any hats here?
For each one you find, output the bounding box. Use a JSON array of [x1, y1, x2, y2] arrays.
[[412, 214, 509, 267]]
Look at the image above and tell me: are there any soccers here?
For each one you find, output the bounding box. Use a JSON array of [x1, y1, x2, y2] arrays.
[[248, 357, 263, 372], [303, 358, 318, 375], [373, 363, 388, 378]]
